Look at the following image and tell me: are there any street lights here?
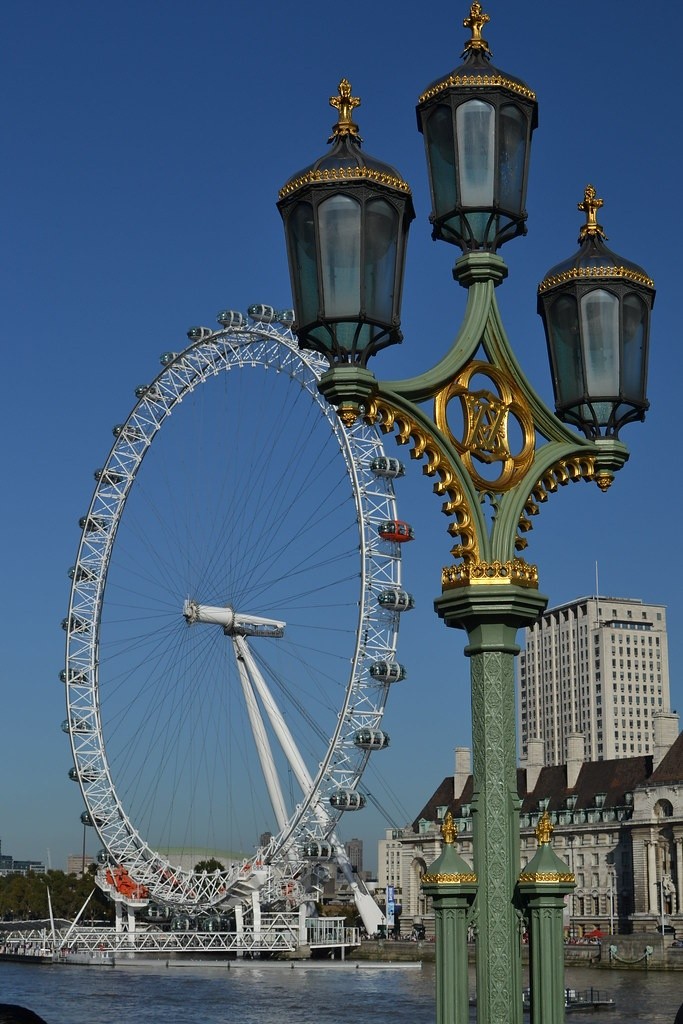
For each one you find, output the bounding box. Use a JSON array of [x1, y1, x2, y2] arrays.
[[381, 916, 384, 938], [276, 1, 656, 1024], [661, 874, 672, 936], [610, 876, 619, 935]]
